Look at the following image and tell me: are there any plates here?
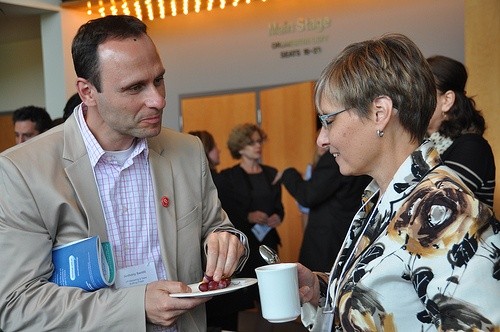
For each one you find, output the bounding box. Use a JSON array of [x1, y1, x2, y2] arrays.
[[169, 278, 258, 298]]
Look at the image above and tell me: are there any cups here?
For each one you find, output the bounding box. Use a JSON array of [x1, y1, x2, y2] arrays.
[[255, 263, 302, 323]]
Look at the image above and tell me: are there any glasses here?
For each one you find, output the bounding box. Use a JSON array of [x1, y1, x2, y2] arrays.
[[318, 107, 352, 128]]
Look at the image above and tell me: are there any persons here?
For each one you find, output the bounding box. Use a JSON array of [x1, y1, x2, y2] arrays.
[[424, 55, 496, 212], [295, 30, 500, 332], [13, 106, 53, 145], [213, 124, 284, 307], [189, 130, 220, 183], [272, 114, 372, 274], [0, 14, 250, 332]]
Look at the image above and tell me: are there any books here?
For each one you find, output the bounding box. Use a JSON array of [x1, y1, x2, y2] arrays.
[[50, 234, 118, 291]]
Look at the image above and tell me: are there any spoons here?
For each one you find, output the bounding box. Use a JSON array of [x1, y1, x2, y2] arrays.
[[259, 244, 281, 264]]
